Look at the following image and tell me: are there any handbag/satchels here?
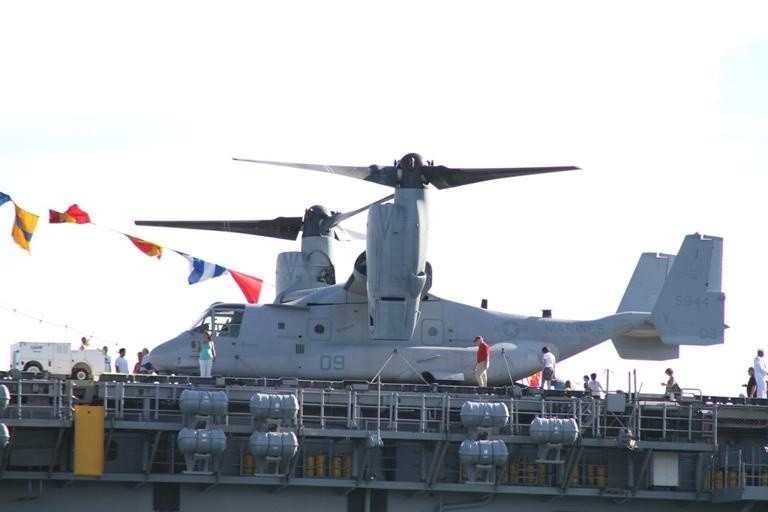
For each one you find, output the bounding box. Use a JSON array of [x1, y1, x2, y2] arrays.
[[550, 379, 559, 386], [672, 382, 682, 397]]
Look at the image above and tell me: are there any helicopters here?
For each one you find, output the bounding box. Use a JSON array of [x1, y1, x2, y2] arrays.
[[132, 147, 731, 391]]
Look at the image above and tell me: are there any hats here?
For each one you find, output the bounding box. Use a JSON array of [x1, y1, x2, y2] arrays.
[[472, 336, 484, 342]]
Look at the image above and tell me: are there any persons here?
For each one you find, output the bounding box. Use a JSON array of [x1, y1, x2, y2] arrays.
[[540, 346, 556, 390], [103, 346, 111, 372], [753, 349, 768, 398], [741, 367, 757, 398], [80, 337, 88, 350], [472, 335, 490, 387], [661, 368, 681, 399], [132, 352, 143, 374], [563, 373, 605, 400], [195, 330, 217, 377], [114, 348, 129, 373], [139, 348, 150, 373]]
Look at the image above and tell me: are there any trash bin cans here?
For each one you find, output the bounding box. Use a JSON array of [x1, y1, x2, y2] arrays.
[[74, 406, 104, 475]]
